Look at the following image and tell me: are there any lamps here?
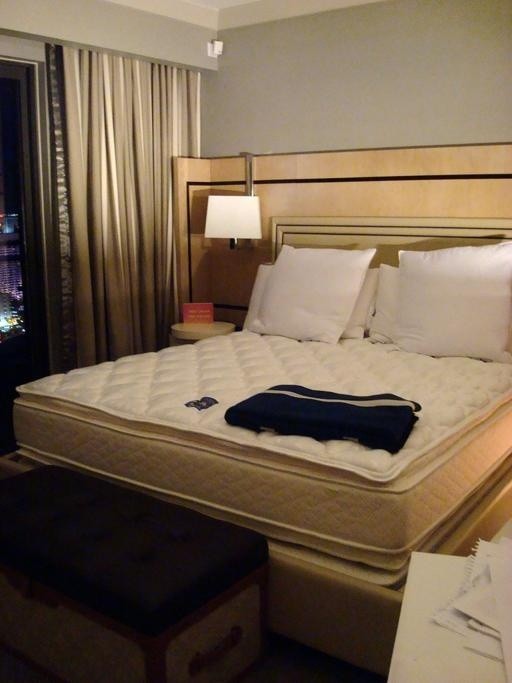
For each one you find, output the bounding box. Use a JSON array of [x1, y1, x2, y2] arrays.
[[203, 196, 263, 252]]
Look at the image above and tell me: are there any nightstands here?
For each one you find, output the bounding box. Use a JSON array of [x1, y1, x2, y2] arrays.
[[168, 321, 235, 348]]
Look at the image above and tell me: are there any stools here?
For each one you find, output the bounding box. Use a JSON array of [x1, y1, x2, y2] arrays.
[[0, 465, 272, 683]]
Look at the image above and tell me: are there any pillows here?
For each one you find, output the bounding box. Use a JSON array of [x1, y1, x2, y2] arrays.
[[242, 241, 512, 363]]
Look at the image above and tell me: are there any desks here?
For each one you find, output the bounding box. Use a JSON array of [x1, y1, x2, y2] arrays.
[[384, 551, 512, 683]]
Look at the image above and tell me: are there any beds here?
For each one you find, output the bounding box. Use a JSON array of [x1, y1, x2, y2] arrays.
[[1, 214, 512, 677]]
